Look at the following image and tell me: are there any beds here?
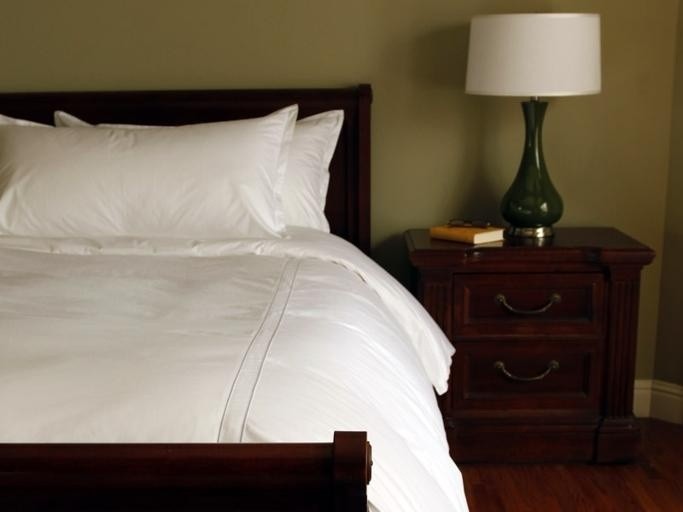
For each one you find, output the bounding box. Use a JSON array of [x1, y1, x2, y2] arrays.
[[0, 82, 470, 512]]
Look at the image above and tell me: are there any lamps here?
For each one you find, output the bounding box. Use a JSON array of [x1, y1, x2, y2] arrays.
[[464, 11, 604, 240]]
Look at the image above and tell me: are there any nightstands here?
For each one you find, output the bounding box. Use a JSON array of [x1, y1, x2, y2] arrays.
[[402, 224, 658, 465]]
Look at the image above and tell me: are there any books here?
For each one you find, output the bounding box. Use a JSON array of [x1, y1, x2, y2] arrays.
[[429, 223, 506, 245]]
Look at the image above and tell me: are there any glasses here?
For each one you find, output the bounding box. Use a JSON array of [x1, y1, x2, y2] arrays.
[[447, 217, 494, 228]]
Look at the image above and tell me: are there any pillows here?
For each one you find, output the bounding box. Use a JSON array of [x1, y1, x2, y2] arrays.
[[51, 107, 345, 235], [0, 101, 300, 240]]
